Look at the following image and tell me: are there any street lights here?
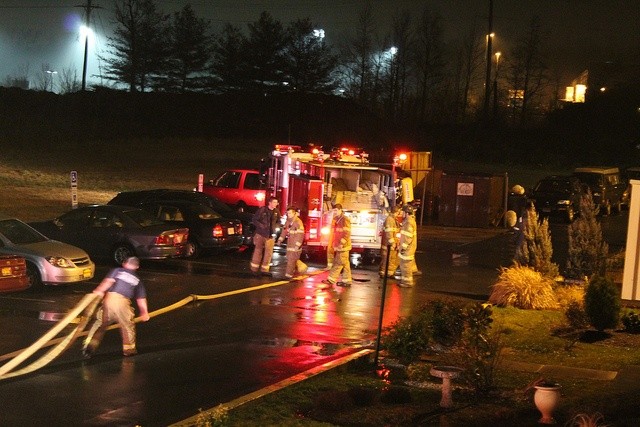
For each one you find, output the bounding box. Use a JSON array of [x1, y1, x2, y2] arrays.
[[46, 69, 58, 92]]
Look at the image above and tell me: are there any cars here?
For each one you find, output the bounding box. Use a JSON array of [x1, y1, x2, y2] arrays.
[[121, 198, 244, 259], [1, 251, 31, 294], [624, 167, 640, 182], [37, 203, 190, 267], [1, 215, 97, 290]]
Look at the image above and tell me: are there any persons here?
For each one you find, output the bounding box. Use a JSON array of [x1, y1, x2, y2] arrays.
[[325, 211, 345, 273], [378, 206, 397, 279], [249, 196, 278, 277], [397, 205, 418, 288], [278, 206, 307, 279], [394, 207, 422, 276], [321, 204, 352, 287], [81, 256, 150, 359]]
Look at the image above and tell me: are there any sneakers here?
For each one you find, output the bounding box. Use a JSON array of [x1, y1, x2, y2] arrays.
[[337, 281, 351, 286], [322, 279, 334, 284], [124, 348, 139, 355], [400, 283, 413, 287], [413, 270, 423, 275]]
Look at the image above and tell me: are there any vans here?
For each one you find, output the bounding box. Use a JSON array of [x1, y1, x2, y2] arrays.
[[574, 166, 622, 216], [104, 187, 260, 247]]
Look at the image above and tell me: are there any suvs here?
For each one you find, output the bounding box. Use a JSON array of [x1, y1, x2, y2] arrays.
[[527, 173, 592, 224], [191, 168, 267, 213]]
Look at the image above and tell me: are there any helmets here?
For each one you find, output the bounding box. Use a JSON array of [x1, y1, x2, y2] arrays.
[[402, 204, 414, 212]]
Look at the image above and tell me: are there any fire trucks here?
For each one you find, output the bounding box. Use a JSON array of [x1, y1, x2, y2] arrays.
[[265, 143, 410, 265]]
